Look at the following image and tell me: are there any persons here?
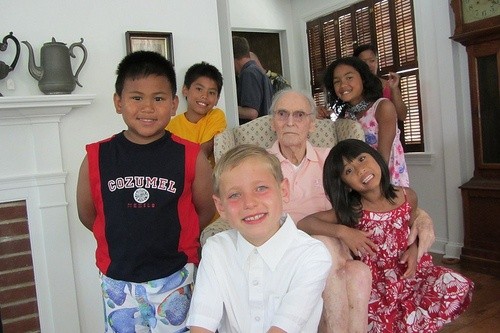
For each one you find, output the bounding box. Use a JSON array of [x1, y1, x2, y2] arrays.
[[164, 62, 228, 178], [231, 35, 292, 125], [186, 144, 333, 333], [76, 50, 215, 333], [256, 88, 433, 333], [234, 52, 291, 90], [353, 43, 407, 121], [315, 56, 409, 187], [296, 138, 475, 333]]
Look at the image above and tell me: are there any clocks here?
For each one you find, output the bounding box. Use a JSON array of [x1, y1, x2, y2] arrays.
[[448, 0, 500, 46]]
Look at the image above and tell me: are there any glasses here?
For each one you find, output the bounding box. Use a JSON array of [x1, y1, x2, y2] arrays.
[[273, 111, 312, 122]]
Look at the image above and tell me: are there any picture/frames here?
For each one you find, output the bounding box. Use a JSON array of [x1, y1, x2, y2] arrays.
[[125, 31, 174, 67]]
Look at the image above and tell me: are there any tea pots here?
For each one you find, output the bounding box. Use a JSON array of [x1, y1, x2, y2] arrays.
[[21, 37, 88, 95]]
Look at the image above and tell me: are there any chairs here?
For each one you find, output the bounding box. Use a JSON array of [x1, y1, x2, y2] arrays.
[[200, 114, 365, 248]]
